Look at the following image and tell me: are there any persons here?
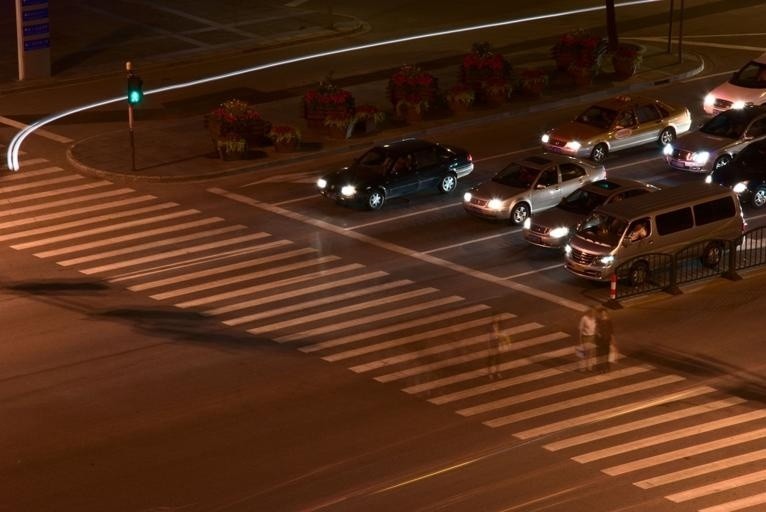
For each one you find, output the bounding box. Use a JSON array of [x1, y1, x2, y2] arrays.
[[486, 312, 504, 381], [577, 305, 597, 374], [595, 307, 617, 374], [628, 222, 647, 241]]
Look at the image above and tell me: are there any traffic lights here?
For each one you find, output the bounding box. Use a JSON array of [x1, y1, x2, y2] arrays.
[[127, 78, 143, 104]]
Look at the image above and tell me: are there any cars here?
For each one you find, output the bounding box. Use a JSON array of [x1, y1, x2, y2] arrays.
[[702, 55, 766, 115], [541, 95, 693, 163], [317, 138, 473, 210], [702, 139, 766, 208], [518, 178, 664, 249], [462, 152, 611, 225], [662, 103, 766, 173]]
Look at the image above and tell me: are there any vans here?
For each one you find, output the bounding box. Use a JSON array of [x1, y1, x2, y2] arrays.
[[560, 182, 749, 286]]
